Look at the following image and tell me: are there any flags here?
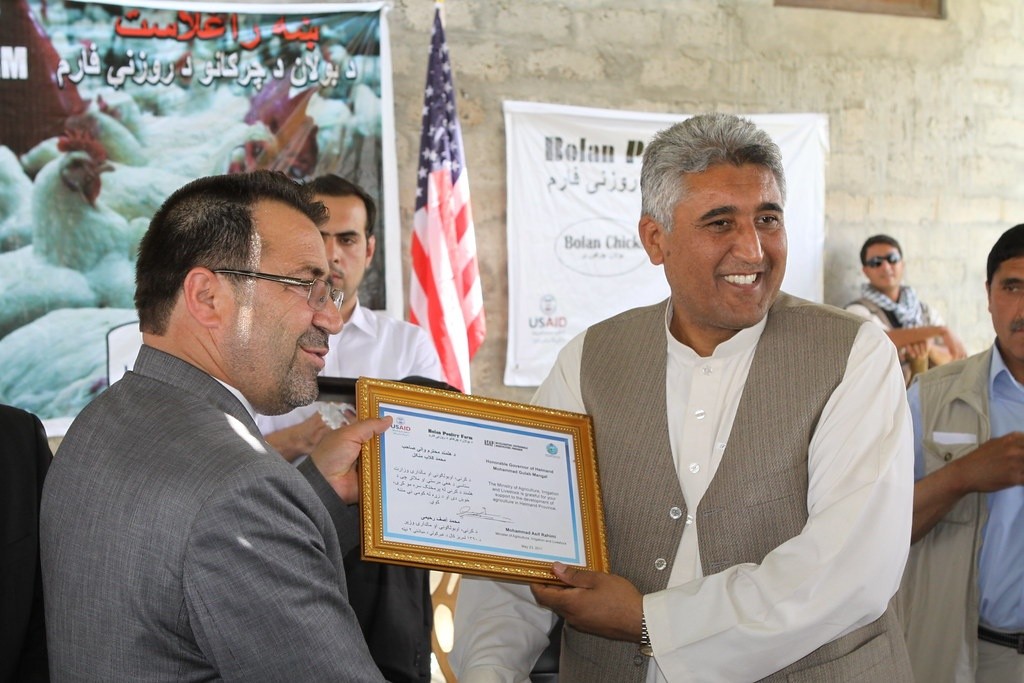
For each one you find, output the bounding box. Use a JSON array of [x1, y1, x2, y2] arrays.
[[409, 6, 487, 397]]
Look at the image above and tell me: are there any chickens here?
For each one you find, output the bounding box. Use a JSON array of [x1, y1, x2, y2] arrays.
[[0, 0, 384, 422]]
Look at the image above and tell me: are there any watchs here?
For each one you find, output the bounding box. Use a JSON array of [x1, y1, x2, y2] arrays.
[[640, 614, 654, 658]]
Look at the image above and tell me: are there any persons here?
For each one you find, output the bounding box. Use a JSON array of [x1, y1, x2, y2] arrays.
[[846, 233, 967, 382], [260, 179, 448, 683], [41, 173, 392, 683], [455, 113, 916, 682], [893, 223, 1023, 683], [0, 404, 53, 683]]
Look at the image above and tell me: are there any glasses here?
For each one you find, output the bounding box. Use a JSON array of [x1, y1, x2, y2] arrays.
[[865, 253, 901, 267], [212, 269, 345, 312]]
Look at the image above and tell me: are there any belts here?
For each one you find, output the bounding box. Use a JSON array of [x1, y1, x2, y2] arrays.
[[977, 625, 1024, 654]]
